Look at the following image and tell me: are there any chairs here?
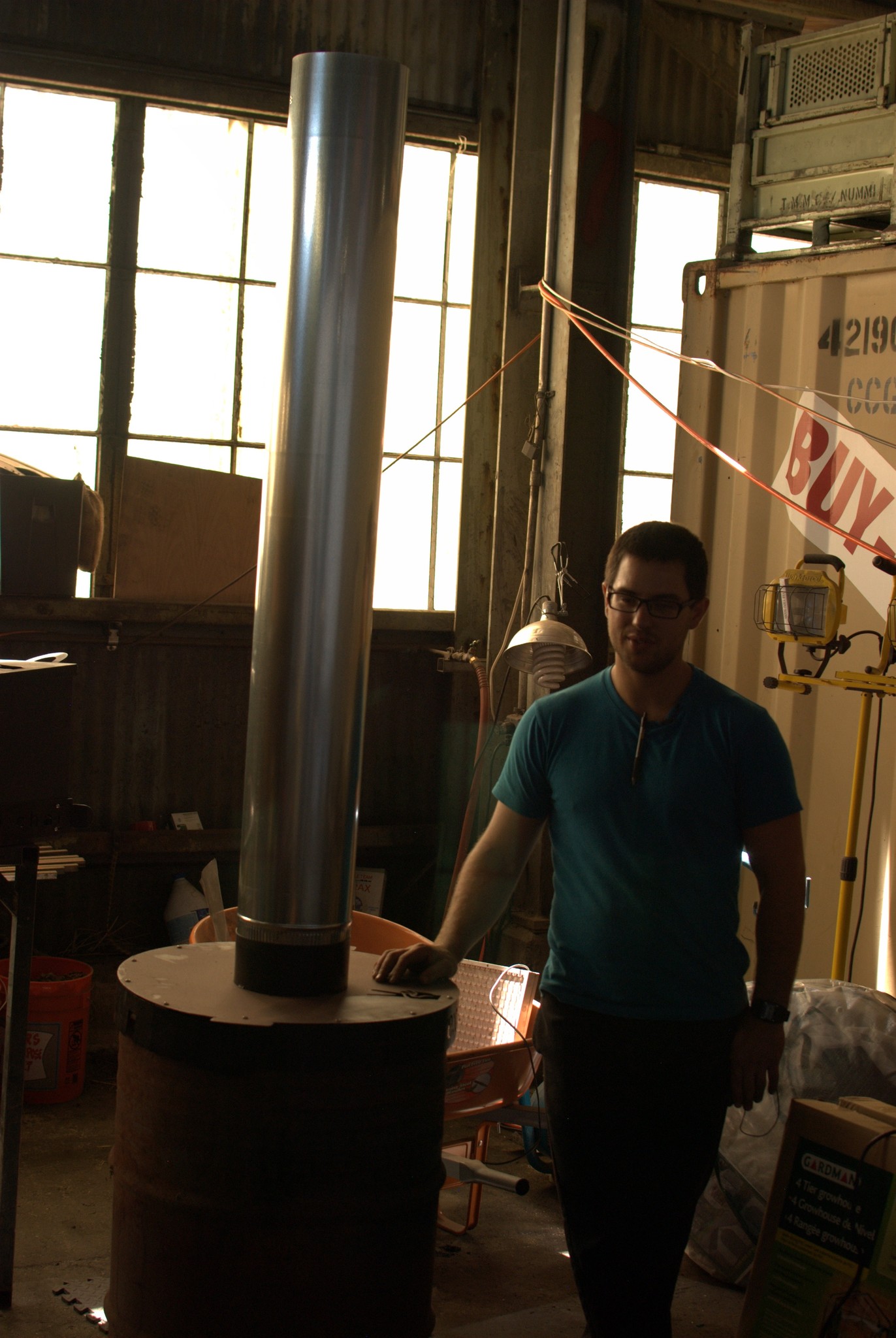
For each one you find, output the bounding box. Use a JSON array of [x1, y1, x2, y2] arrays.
[[190, 898, 541, 1233]]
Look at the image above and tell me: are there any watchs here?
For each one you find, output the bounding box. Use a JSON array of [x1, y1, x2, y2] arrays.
[[749, 998, 791, 1024]]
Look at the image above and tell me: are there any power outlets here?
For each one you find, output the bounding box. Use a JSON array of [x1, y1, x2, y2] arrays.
[[507, 714, 523, 729]]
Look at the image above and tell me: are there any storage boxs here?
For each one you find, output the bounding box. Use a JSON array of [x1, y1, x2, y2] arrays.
[[1, 476, 87, 598], [739, 1095, 895, 1336]]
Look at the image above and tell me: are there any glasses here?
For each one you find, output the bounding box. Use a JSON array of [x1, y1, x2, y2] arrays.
[[605, 584, 699, 621]]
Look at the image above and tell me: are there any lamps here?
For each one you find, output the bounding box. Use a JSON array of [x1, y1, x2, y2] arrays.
[[501, 598, 593, 692]]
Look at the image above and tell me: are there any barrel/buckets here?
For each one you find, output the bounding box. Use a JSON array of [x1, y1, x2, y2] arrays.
[[0, 956, 93, 1105]]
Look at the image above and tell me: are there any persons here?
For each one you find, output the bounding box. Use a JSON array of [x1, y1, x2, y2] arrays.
[[371, 519, 807, 1338]]
